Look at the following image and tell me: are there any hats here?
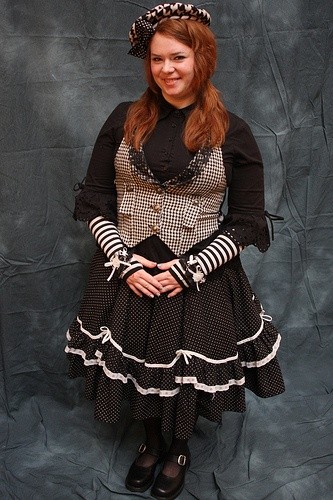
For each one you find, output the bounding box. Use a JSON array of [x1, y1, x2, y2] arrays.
[[128, 1, 213, 59]]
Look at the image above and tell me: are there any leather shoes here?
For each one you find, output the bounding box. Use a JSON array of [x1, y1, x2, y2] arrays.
[[150, 444, 191, 500], [123, 443, 160, 493]]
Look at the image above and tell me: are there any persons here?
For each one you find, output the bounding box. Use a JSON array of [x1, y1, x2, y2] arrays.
[[64, 1, 285, 498]]
